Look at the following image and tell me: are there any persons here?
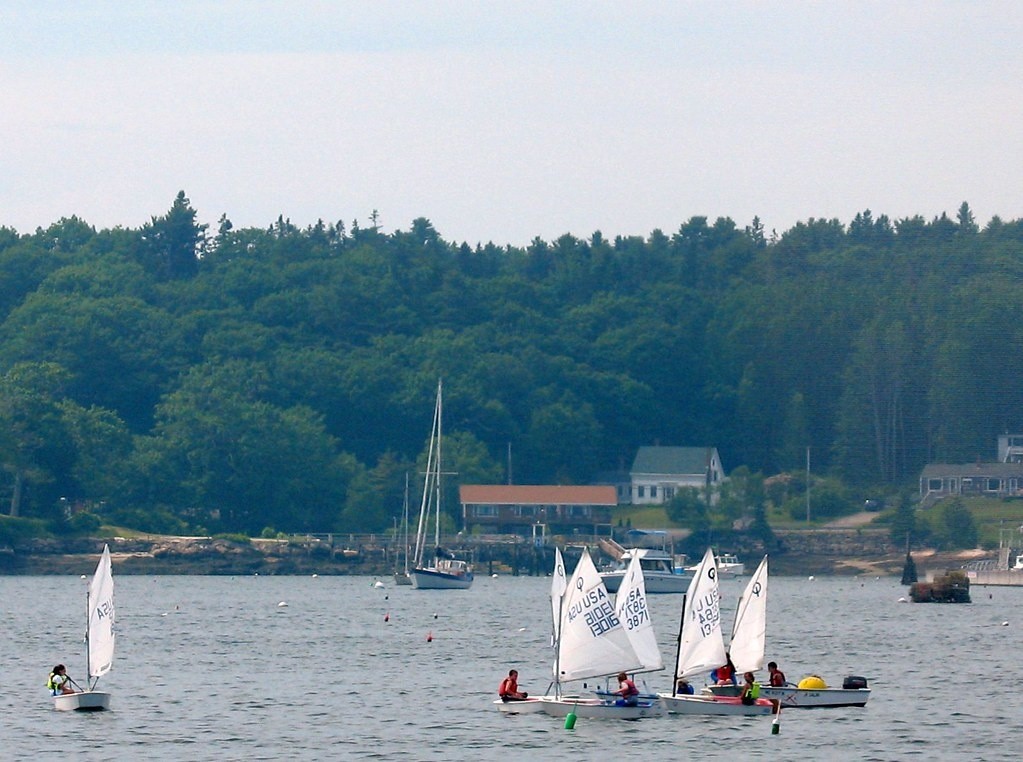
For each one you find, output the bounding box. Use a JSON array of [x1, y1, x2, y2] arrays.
[[676, 678, 695, 695], [709, 652, 740, 693], [498, 670, 528, 703], [609, 672, 640, 708], [767, 661, 790, 688], [48, 664, 75, 697], [740, 671, 756, 706]]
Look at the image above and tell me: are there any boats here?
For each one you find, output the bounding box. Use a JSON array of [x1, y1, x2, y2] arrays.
[[682, 553, 742, 580], [668, 553, 692, 576], [908, 566, 972, 605], [595, 529, 702, 595]]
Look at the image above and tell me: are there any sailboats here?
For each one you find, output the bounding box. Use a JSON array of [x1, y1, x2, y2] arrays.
[[409, 375, 473, 592], [654, 545, 774, 717], [723, 555, 871, 709], [493, 545, 584, 713], [389, 471, 421, 586], [51, 540, 121, 714], [537, 544, 672, 722]]
